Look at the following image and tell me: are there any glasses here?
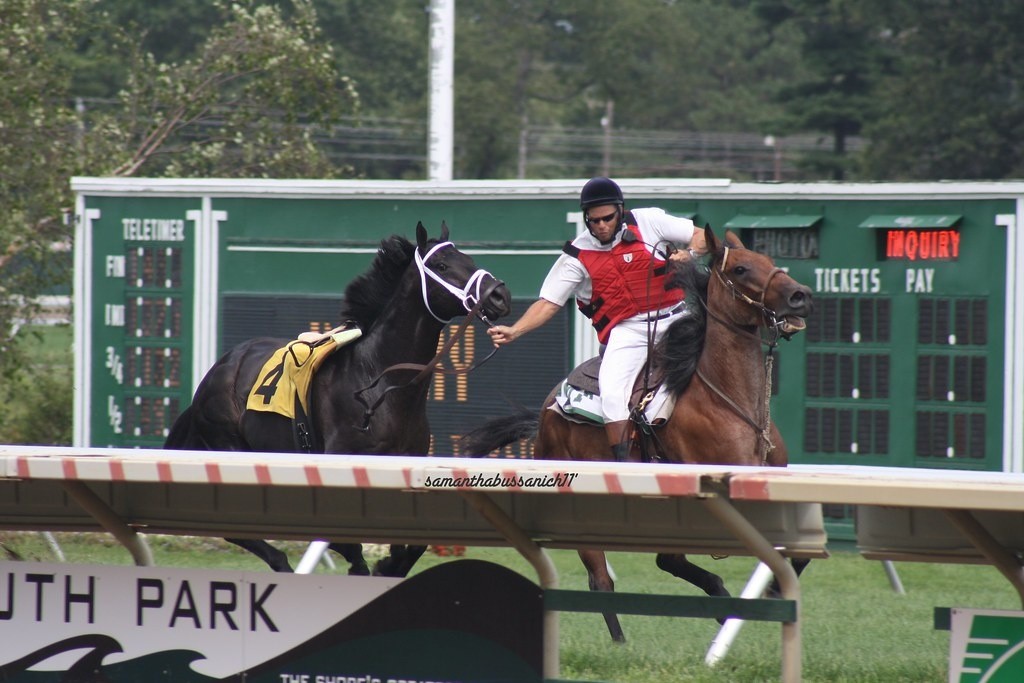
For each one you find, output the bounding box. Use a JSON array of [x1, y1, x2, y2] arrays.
[[587, 210, 618, 223]]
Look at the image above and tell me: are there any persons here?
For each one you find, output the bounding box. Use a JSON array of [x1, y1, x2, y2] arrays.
[[483, 176, 716, 473]]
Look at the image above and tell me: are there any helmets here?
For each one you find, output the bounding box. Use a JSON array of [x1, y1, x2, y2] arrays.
[[579, 176, 624, 209]]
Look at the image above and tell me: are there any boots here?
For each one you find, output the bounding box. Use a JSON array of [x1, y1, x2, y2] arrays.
[[607, 420, 641, 463]]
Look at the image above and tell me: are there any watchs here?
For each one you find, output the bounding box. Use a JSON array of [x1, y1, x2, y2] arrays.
[[684, 246, 700, 261]]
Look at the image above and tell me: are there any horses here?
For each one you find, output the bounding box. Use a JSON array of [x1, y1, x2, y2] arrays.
[[453, 221, 823, 643], [162, 218, 514, 574]]
